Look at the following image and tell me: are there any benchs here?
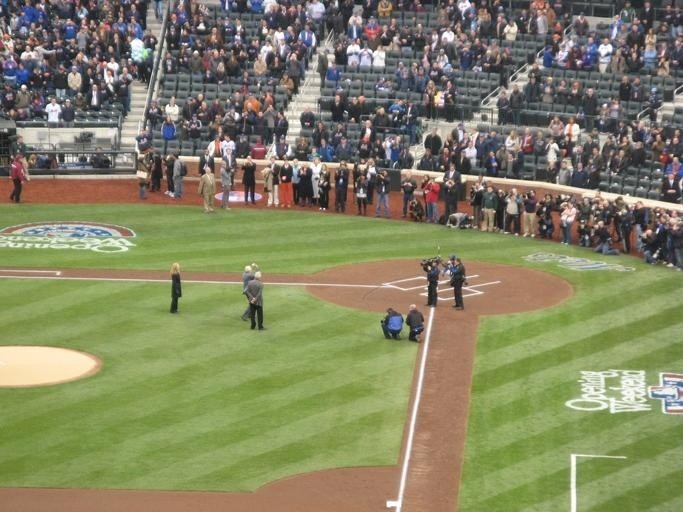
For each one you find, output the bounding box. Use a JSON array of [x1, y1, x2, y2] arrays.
[[16, 0, 683, 203]]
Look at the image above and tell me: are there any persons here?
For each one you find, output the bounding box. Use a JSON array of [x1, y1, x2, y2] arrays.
[[241, 264, 255, 322], [244, 269, 265, 331], [404, 303, 424, 342], [0, 1, 682, 269], [380, 307, 403, 339], [422, 259, 437, 307], [169, 262, 182, 313], [448, 257, 464, 310], [441, 254, 457, 277]]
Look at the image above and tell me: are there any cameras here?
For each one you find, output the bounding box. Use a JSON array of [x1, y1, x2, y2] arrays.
[[510, 191, 513, 196]]
